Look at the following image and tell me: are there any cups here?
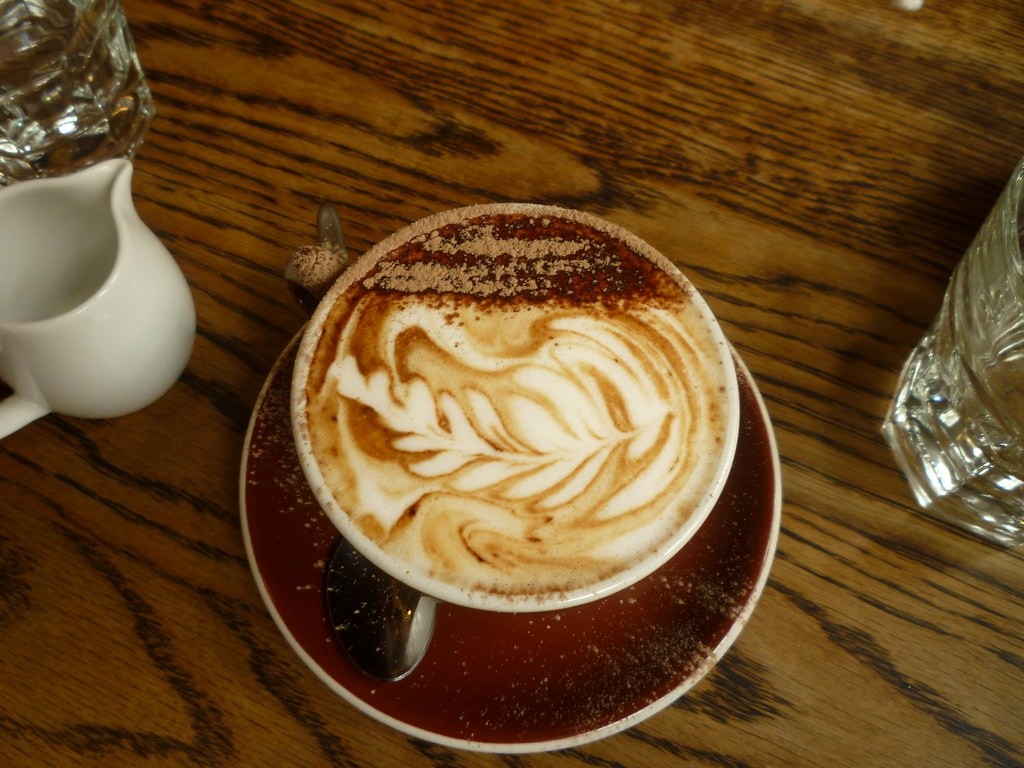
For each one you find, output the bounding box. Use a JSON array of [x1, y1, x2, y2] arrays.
[[880, 150, 1023, 548], [292, 202, 741, 614], [0, 0, 158, 179]]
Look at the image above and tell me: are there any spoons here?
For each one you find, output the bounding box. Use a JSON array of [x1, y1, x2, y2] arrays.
[[325, 538, 437, 683]]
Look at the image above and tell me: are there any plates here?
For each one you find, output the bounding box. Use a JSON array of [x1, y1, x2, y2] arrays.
[[239, 321, 783, 755]]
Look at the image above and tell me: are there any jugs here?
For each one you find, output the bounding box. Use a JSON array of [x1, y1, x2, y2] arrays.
[[0, 157, 197, 441]]
